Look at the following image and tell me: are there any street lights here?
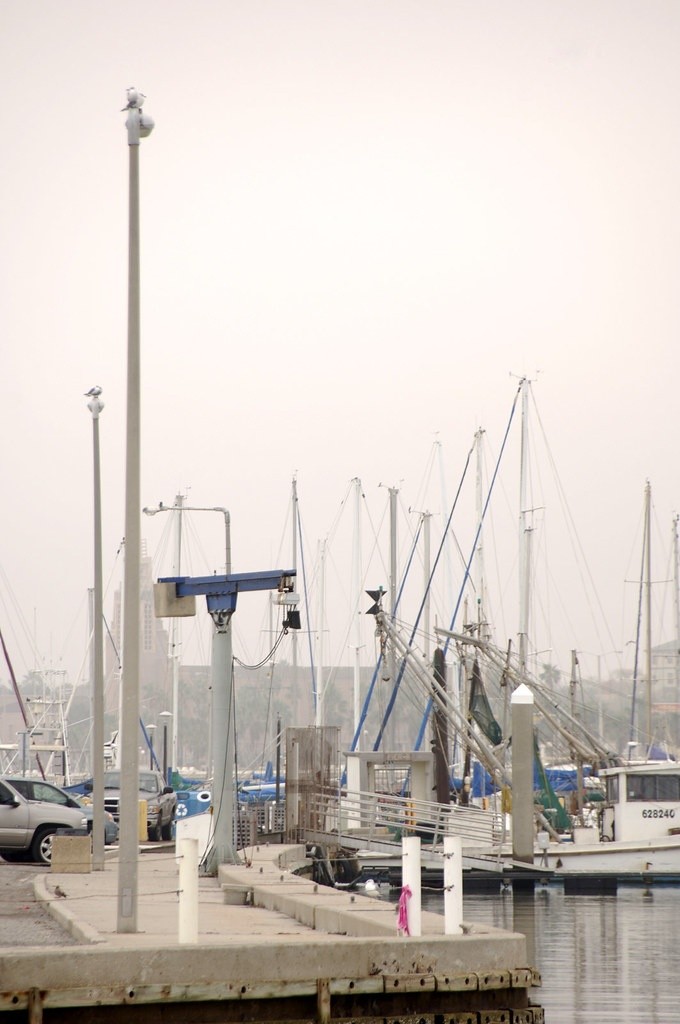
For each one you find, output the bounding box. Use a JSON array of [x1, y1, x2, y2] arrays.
[[83, 384, 107, 874], [120, 83, 155, 937], [145, 725, 157, 770], [261, 629, 330, 726], [507, 649, 551, 683], [575, 650, 622, 747], [157, 710, 173, 785]]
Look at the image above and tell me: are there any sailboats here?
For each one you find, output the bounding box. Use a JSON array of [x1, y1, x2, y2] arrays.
[[39, 373, 680, 887]]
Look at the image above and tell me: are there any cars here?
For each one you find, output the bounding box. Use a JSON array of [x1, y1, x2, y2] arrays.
[[7, 779, 118, 853], [0, 779, 89, 865], [85, 771, 178, 841]]
